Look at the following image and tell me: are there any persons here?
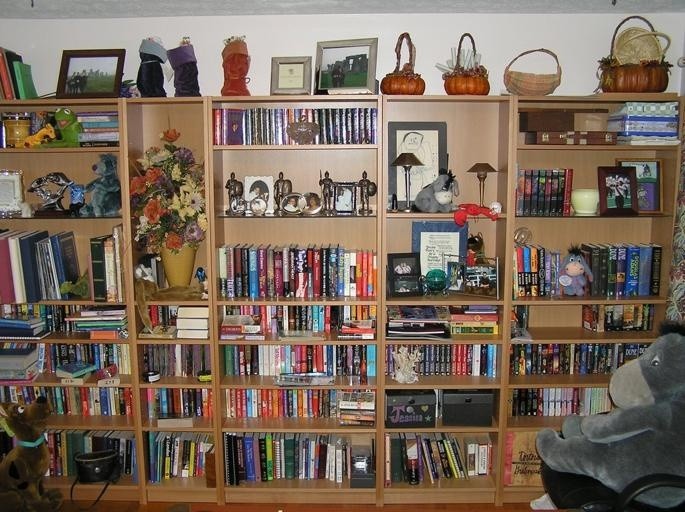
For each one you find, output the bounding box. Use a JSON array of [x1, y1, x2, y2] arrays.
[[606, 175, 631, 208], [65, 69, 88, 94], [331, 66, 345, 88], [226, 170, 377, 217]]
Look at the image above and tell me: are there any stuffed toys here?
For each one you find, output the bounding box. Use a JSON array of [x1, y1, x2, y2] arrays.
[[0, 396, 65, 512], [77, 154, 121, 218], [536, 321, 685, 509], [413, 168, 459, 213]]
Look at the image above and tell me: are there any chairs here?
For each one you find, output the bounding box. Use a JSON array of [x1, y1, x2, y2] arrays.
[[539, 412, 684, 512]]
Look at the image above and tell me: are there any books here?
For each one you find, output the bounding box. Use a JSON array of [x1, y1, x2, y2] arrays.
[[213, 106, 377, 147], [517, 167, 573, 217], [607, 102, 682, 147], [0, 109, 120, 147], [0, 46, 39, 102]]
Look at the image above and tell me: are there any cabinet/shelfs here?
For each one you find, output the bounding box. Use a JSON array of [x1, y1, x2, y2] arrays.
[[382, 92, 514, 508], [0, 97, 143, 508], [208, 95, 381, 507], [121, 94, 222, 507], [498, 92, 682, 506]]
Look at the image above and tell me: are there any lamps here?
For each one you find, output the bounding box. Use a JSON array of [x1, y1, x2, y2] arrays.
[[466, 162, 499, 206], [390, 152, 424, 207]]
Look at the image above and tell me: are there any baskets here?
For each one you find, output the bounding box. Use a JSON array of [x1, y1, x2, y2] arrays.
[[600, 16, 671, 92], [380, 32, 425, 94], [505, 49, 561, 96], [444, 32, 490, 94]]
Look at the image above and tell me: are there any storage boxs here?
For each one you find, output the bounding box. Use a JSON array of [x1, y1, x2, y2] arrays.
[[440, 390, 495, 427], [384, 389, 437, 429]]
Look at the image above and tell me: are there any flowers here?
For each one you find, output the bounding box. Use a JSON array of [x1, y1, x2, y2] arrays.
[[128, 128, 208, 255]]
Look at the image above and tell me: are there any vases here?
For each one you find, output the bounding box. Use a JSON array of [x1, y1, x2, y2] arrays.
[[159, 246, 200, 289]]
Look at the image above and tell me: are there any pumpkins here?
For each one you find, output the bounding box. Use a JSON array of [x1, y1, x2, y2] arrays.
[[601, 65, 669, 93], [380, 71, 425, 95], [444, 74, 490, 95]]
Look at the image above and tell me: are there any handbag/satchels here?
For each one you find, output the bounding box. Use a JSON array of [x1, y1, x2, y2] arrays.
[[70, 443, 122, 511]]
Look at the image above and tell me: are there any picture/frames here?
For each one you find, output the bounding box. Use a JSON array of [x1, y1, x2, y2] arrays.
[[411, 220, 469, 293], [596, 165, 639, 217], [0, 169, 26, 220], [313, 36, 379, 95], [614, 157, 665, 215], [55, 47, 134, 101], [387, 121, 448, 212], [229, 174, 357, 216], [269, 55, 312, 96], [385, 252, 422, 298]]
